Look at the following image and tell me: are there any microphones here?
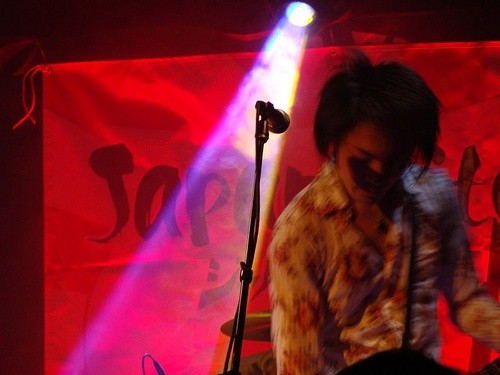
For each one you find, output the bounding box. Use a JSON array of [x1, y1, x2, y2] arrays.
[[256, 100, 290, 133]]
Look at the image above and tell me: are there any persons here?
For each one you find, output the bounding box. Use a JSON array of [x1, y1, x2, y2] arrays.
[[268, 49, 499, 375]]
